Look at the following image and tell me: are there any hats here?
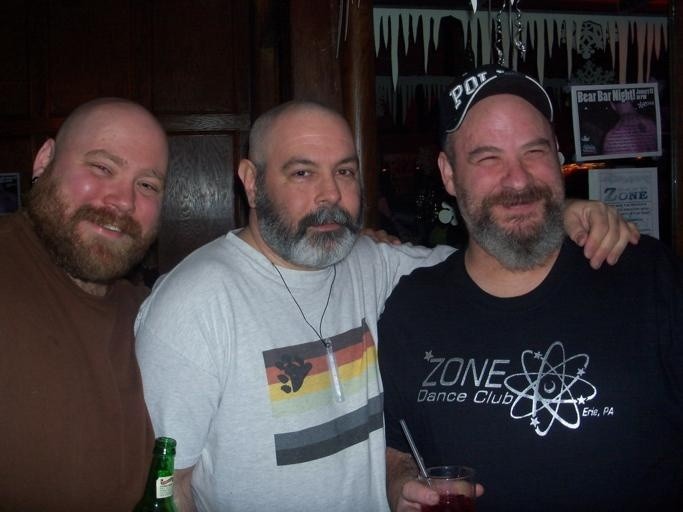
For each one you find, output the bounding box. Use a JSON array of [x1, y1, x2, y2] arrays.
[[440, 63, 554, 136]]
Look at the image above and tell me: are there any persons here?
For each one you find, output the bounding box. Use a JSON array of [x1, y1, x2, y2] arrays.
[[1, 96, 402, 511], [132, 99, 640, 512], [603, 98, 656, 154], [377, 131, 420, 242], [378, 68, 681, 510]]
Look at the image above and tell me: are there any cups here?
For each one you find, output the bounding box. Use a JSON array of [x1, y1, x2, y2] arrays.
[[418, 465, 478, 512]]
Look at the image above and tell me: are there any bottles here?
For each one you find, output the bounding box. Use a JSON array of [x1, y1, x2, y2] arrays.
[[132, 436, 178, 512]]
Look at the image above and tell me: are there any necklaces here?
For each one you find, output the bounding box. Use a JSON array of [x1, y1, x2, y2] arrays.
[[258, 252, 337, 348]]
